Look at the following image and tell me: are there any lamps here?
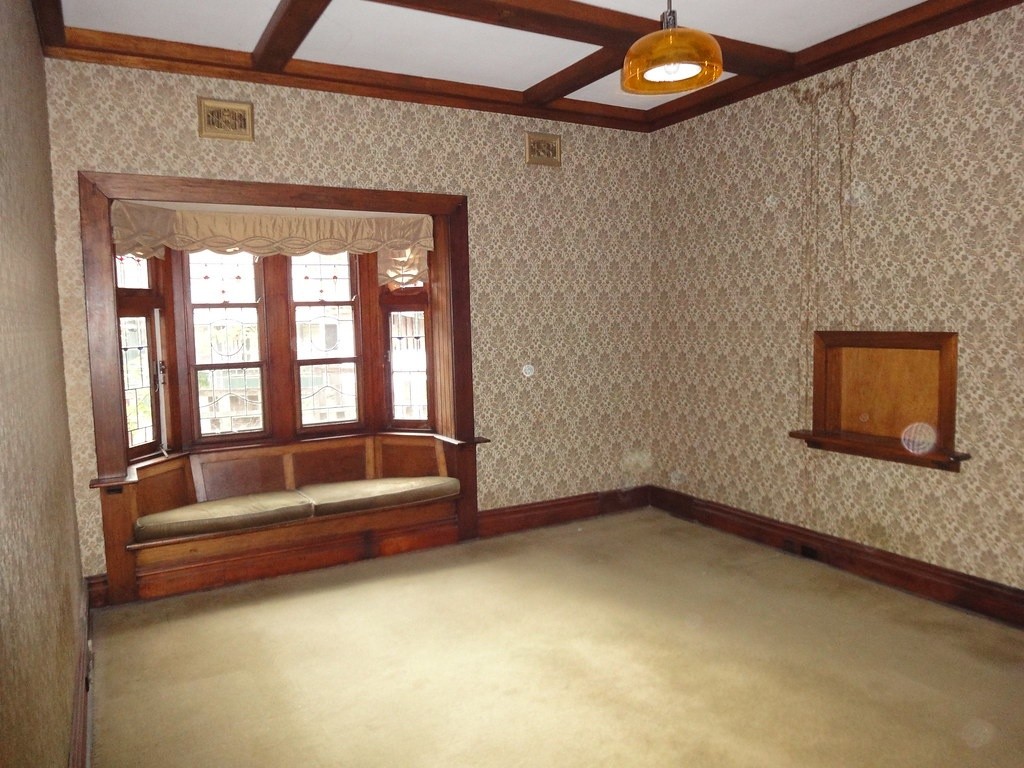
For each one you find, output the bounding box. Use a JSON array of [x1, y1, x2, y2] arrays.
[[620, 1, 722, 94]]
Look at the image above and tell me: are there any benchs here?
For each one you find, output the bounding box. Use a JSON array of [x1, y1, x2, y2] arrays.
[[125, 478, 460, 601]]
